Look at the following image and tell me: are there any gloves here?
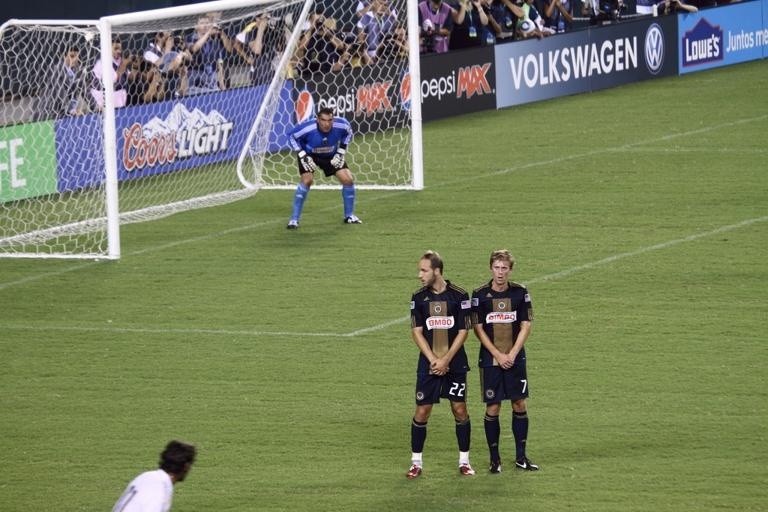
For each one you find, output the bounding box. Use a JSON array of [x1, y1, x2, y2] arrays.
[[298, 149, 317, 172], [330, 147, 346, 169]]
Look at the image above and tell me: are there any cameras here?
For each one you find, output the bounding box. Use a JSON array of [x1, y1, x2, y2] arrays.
[[173, 37, 181, 45], [315, 16, 326, 31], [213, 19, 224, 30]]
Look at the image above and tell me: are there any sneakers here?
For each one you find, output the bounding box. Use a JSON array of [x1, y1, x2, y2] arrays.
[[343, 214, 362, 224], [460, 462, 474, 475], [406, 464, 422, 479], [489, 458, 501, 473], [287, 219, 298, 230], [516, 456, 538, 471]]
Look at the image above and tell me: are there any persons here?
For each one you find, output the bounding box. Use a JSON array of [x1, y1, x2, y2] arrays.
[[405, 249, 476, 480], [111, 439, 197, 512], [418, 1, 701, 55], [286, 108, 363, 230], [469, 249, 538, 474], [44, 1, 409, 120]]
